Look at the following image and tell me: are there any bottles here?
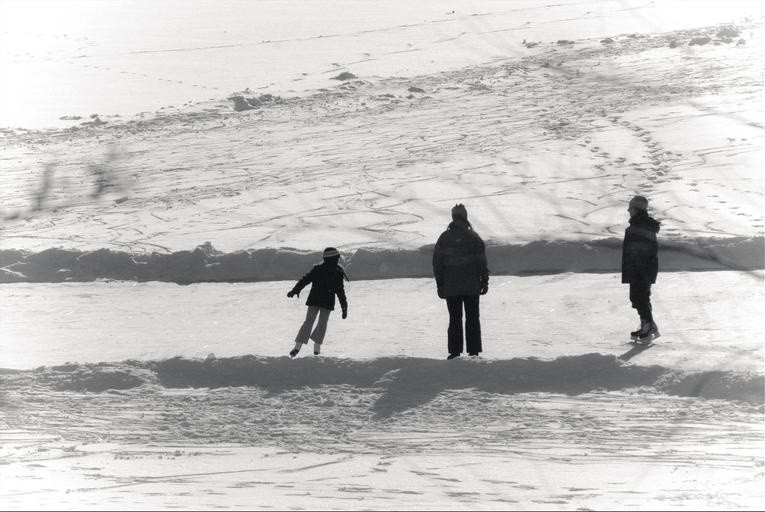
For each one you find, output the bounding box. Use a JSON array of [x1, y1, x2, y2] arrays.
[[451, 203, 467, 222], [628, 196, 648, 211], [323, 247, 341, 262]]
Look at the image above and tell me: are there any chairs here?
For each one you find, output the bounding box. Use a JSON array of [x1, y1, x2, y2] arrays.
[[631, 319, 658, 339], [468, 351, 478, 356], [447, 352, 460, 360]]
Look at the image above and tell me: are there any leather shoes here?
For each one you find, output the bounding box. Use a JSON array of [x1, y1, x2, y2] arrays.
[[438, 286, 444, 299], [480, 278, 488, 295], [286, 289, 300, 299], [341, 307, 347, 319]]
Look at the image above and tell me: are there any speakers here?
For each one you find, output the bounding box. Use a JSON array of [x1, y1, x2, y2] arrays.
[[314, 343, 321, 355], [289, 342, 303, 357]]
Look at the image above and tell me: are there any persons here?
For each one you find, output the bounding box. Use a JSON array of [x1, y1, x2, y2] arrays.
[[432, 203, 490, 360], [287, 247, 349, 357], [621, 195, 660, 339]]
